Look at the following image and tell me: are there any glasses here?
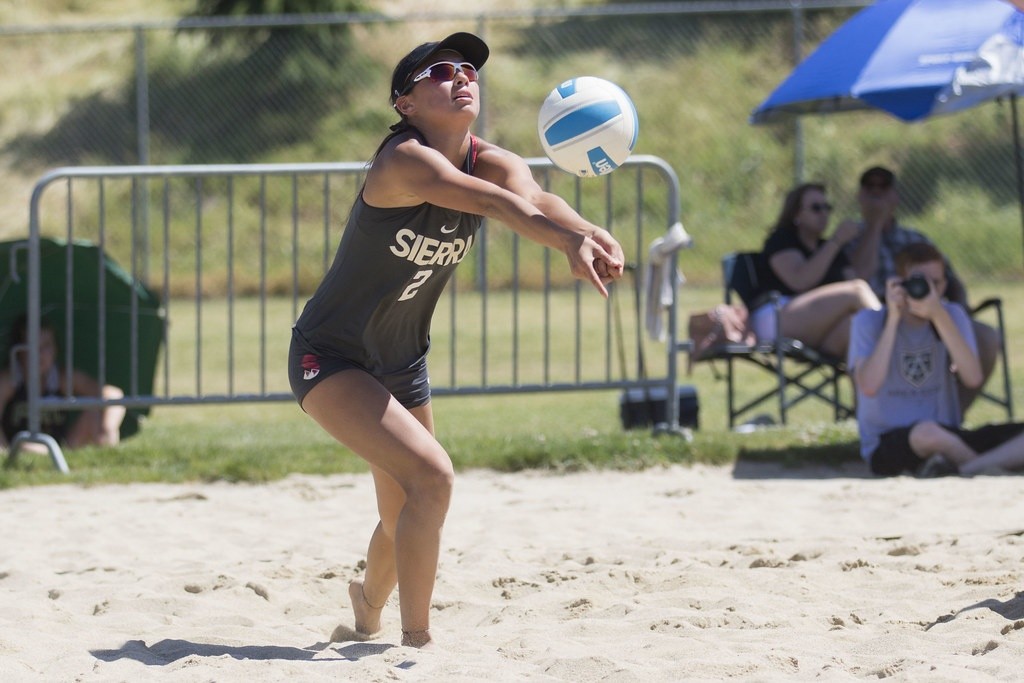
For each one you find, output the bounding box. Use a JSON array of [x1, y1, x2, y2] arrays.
[[802, 203, 834, 215], [400, 61, 478, 98]]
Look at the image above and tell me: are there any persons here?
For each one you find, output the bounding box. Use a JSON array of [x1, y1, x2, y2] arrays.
[[829, 167, 1001, 424], [0, 315, 125, 455], [288, 33, 624, 645], [847, 244, 1024, 478], [752, 185, 881, 357]]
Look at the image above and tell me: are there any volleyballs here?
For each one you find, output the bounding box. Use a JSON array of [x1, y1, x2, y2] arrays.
[[538, 75, 642, 178]]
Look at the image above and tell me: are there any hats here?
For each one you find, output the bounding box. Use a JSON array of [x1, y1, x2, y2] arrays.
[[392, 31, 489, 105], [860, 168, 897, 191]]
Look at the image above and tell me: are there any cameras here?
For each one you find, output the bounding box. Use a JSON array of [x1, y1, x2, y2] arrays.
[[900, 273, 929, 299]]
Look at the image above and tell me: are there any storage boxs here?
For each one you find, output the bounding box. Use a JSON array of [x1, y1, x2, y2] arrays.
[[621, 382, 701, 433]]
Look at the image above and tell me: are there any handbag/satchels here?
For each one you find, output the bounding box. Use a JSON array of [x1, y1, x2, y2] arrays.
[[689, 308, 761, 354]]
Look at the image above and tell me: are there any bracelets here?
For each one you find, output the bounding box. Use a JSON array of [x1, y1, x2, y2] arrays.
[[834, 236, 843, 248]]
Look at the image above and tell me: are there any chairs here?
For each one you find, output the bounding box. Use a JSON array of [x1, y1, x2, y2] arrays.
[[676, 252, 1015, 429]]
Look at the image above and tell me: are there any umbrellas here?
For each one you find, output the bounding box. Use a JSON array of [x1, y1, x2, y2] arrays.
[[0, 238, 162, 439], [751, 0, 1024, 229]]
